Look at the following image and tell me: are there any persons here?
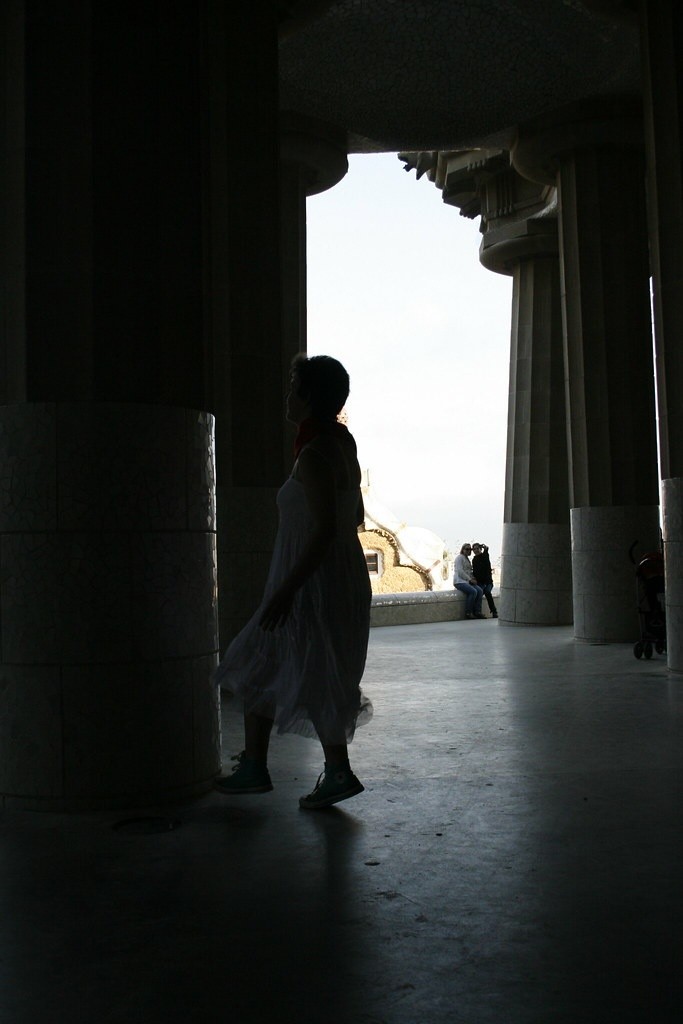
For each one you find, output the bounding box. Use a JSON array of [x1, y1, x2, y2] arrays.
[[632, 547, 666, 660], [210, 355, 372, 806], [454, 542, 498, 618]]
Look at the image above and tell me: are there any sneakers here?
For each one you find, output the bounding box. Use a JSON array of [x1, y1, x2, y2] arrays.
[[213, 750, 274, 794], [299, 759, 364, 810]]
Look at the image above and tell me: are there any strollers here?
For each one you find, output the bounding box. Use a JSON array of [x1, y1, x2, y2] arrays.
[[628, 540, 667, 660]]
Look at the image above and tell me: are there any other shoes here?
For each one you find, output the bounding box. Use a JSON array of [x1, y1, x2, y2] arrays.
[[475, 612, 487, 619], [464, 613, 477, 619], [493, 613, 498, 618]]
